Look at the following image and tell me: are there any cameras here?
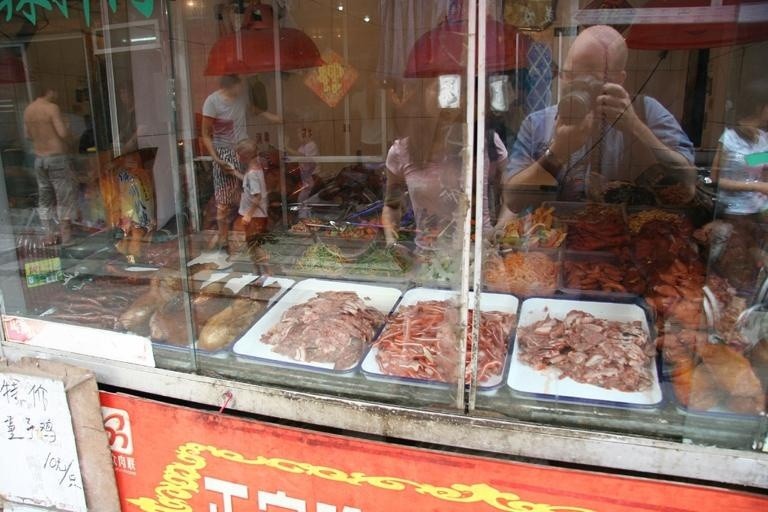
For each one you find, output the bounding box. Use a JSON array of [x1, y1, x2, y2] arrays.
[[557, 74, 605, 126]]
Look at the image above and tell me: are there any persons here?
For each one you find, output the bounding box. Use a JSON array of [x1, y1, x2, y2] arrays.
[[380, 60, 510, 251], [223, 138, 272, 281], [113, 80, 169, 224], [708, 81, 766, 248], [22, 81, 78, 248], [201, 73, 284, 249], [284, 124, 321, 232], [503, 22, 699, 216]]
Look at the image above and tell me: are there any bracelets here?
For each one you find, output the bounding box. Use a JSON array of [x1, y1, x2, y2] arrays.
[[544, 149, 564, 170], [388, 241, 398, 250]]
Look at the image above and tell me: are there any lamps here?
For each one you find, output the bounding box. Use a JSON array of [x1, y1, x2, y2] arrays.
[[401, 1, 558, 79], [200, 0, 329, 78]]
